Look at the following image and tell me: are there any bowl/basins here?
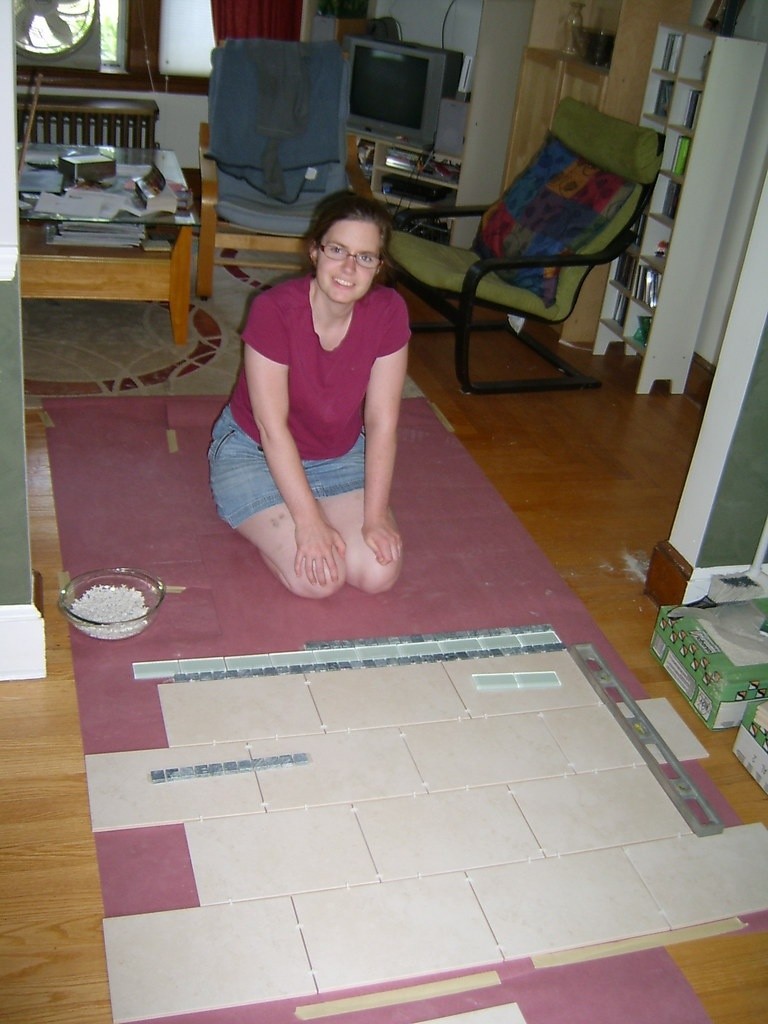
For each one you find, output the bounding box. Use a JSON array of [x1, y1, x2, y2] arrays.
[[56, 568, 165, 639], [571, 27, 618, 68]]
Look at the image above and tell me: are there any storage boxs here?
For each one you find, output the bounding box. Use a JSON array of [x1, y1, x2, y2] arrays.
[[58, 153, 116, 180], [649, 594, 768, 796]]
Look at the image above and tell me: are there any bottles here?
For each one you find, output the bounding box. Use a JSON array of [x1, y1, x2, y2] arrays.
[[560, 2, 587, 52]]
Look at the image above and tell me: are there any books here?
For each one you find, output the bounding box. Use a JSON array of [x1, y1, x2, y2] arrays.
[[605, 212, 662, 326], [652, 32, 705, 130], [661, 181, 680, 218], [134, 163, 179, 215], [46, 222, 146, 248], [667, 134, 692, 178]]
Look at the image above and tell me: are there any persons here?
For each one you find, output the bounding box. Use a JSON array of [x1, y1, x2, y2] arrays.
[[206, 189, 411, 600]]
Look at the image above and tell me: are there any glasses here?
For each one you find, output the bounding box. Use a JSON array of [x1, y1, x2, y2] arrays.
[[317, 240, 382, 268]]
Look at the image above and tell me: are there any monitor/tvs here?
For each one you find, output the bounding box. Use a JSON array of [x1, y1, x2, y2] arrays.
[[338, 34, 448, 153]]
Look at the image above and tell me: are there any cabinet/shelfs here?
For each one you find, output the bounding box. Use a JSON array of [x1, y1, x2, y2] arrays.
[[498, 0, 692, 343], [590, 21, 768, 394], [345, 131, 463, 244]]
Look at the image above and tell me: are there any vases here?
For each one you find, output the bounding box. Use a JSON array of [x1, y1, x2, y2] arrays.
[[560, 3, 614, 68]]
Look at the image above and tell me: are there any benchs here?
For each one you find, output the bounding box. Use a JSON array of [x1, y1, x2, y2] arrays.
[[16, 93, 160, 150]]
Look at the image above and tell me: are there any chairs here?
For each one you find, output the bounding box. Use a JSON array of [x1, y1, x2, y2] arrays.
[[196, 37, 376, 299], [381, 94, 667, 395]]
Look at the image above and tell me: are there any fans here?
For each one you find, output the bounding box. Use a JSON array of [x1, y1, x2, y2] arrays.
[[14, 0, 101, 70]]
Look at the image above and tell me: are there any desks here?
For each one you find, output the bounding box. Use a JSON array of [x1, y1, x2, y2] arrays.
[[16, 142, 202, 346]]
